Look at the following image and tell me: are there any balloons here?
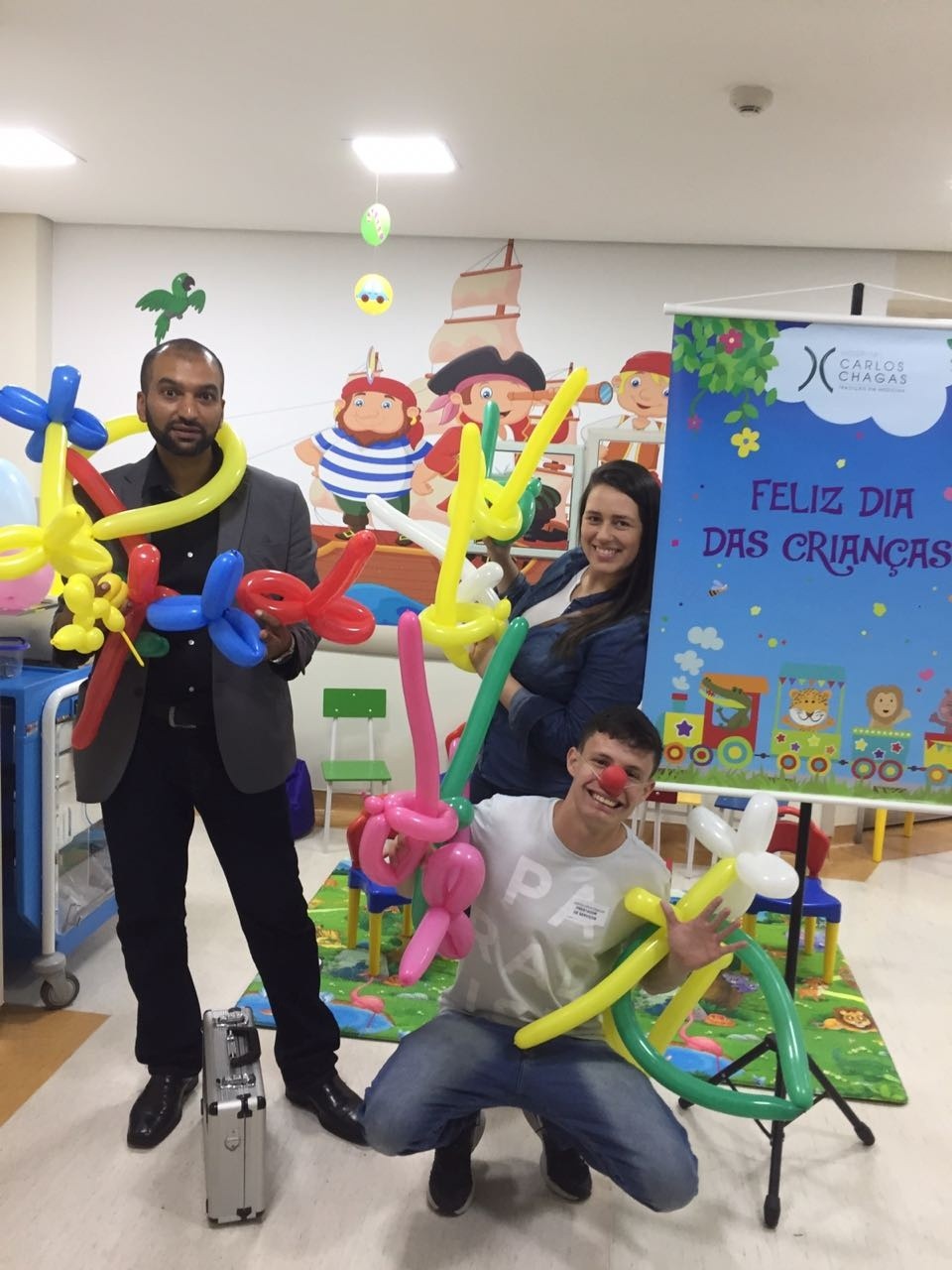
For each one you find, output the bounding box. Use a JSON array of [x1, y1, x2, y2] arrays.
[[349, 366, 593, 988], [0, 363, 374, 749], [513, 796, 817, 1123]]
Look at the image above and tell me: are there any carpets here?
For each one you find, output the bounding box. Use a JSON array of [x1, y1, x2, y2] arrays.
[[237, 858, 910, 1110]]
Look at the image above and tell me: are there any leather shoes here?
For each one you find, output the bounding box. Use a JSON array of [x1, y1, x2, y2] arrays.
[[285, 1075, 373, 1147], [126, 1071, 202, 1149]]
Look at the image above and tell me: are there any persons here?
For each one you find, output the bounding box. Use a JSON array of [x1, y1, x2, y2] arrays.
[[51, 339, 375, 1158], [462, 459, 662, 807], [360, 705, 753, 1218]]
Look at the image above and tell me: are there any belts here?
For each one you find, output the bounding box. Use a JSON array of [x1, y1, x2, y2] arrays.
[[142, 702, 215, 729]]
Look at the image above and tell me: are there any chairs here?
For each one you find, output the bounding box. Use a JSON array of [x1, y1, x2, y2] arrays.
[[322, 687, 394, 835], [740, 807, 841, 989]]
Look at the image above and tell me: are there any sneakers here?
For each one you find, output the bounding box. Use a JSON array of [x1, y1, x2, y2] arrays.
[[424, 1111, 486, 1216], [520, 1107, 592, 1200]]
